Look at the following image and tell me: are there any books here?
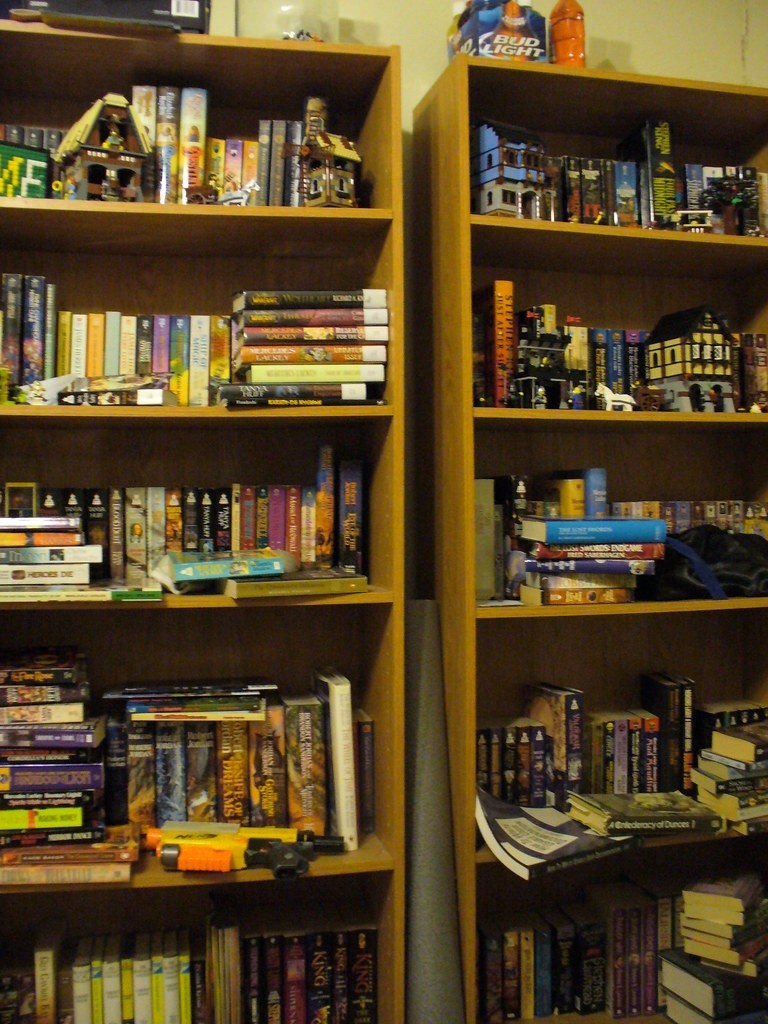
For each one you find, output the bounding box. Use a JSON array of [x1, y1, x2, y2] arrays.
[[473, 670, 767, 880], [471, 278, 768, 408], [2, 87, 303, 206], [477, 866, 768, 1024], [0, 647, 375, 886], [0, 446, 368, 601], [0, 273, 390, 408], [542, 114, 768, 238], [1, 908, 376, 1023], [475, 467, 767, 605]]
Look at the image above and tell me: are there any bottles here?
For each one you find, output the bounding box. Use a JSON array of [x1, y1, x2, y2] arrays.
[[549, 0, 586, 67]]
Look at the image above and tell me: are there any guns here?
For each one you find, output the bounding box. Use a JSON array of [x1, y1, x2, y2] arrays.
[[146, 818, 346, 884]]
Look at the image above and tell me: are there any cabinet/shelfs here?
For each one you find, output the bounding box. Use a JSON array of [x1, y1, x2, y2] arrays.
[[411, 52, 768, 1024], [0, 17, 406, 1024]]
